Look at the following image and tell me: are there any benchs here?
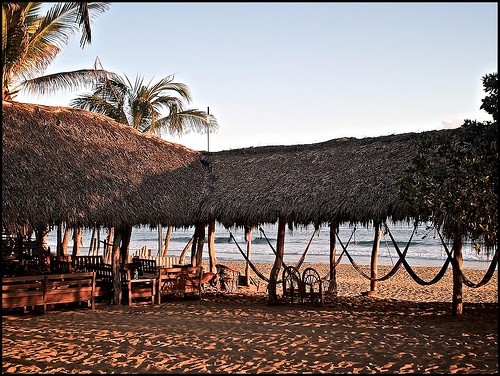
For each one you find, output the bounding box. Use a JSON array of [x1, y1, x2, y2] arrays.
[[1, 251, 208, 317]]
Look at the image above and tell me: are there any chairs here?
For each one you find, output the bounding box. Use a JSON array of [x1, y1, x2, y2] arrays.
[[281, 265, 310, 305], [215, 263, 240, 291], [301, 266, 336, 306]]
